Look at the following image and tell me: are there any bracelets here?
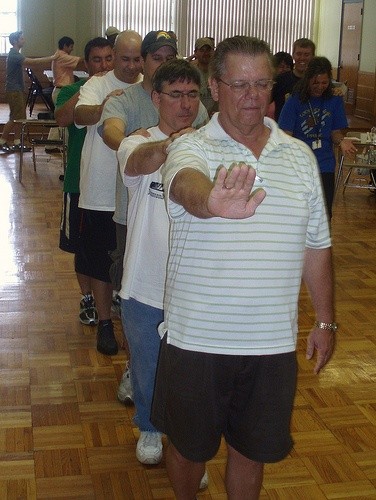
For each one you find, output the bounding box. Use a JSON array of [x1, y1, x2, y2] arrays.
[[337, 136, 345, 145], [313, 321, 338, 333]]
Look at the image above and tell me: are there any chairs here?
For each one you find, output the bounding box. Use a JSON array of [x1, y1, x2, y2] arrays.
[[24, 67, 53, 117], [335, 131, 376, 193], [30, 112, 65, 172]]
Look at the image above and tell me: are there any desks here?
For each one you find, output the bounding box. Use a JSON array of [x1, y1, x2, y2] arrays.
[[13, 118, 59, 184], [345, 115, 375, 132], [43, 70, 89, 82]]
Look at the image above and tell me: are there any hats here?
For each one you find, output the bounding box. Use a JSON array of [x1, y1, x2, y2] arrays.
[[194, 37, 214, 50], [105, 26, 119, 36], [141, 32, 179, 56]]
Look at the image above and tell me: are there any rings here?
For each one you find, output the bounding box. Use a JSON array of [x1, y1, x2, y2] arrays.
[[223, 184, 232, 189]]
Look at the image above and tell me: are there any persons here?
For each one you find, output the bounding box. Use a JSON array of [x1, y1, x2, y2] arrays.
[[44, 25, 144, 355], [270, 36, 360, 228], [182, 36, 219, 120], [0, 31, 61, 153], [116, 59, 209, 489], [152, 35, 336, 500], [96, 29, 209, 402]]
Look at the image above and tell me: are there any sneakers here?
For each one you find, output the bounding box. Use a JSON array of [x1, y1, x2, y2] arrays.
[[111, 292, 121, 318], [96, 319, 119, 355], [79, 294, 98, 325], [136, 430, 163, 465]]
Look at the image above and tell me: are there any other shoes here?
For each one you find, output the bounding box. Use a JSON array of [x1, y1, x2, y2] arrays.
[[117, 370, 134, 405], [12, 143, 32, 152], [0, 143, 13, 152], [45, 147, 63, 153]]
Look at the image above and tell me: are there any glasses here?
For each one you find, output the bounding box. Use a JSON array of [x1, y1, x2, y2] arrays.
[[159, 90, 200, 100], [216, 73, 274, 94]]
